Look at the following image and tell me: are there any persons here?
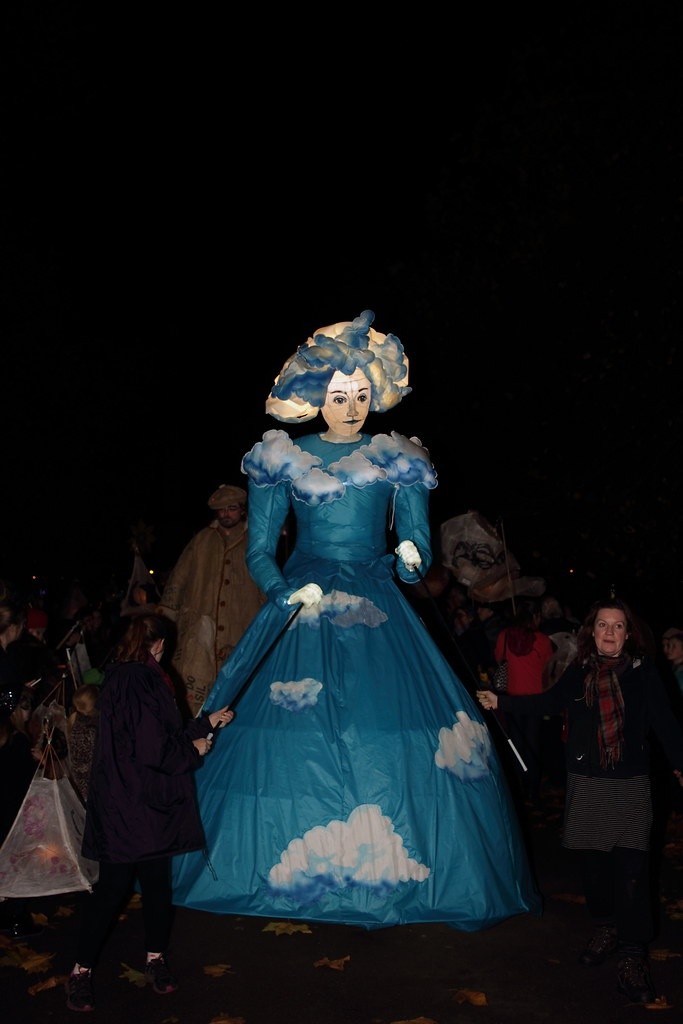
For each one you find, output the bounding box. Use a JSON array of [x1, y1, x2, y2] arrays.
[[546, 594, 677, 1009], [63, 609, 236, 1017], [0, 482, 682, 941], [133, 306, 541, 928]]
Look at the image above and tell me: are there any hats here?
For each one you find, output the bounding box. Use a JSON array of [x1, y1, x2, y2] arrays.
[[208, 485, 247, 508], [27, 608, 48, 628], [57, 589, 88, 607], [0, 684, 24, 723]]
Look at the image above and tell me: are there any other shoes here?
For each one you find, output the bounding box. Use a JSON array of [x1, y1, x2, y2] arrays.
[[518, 796, 540, 810]]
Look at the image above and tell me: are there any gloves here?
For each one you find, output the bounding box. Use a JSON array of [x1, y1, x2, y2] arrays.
[[288, 583, 324, 607], [395, 540, 421, 572]]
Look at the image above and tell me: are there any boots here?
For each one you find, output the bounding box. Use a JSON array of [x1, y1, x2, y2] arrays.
[[1, 900, 16, 932], [616, 951, 669, 1009], [577, 925, 617, 971], [11, 910, 46, 940]]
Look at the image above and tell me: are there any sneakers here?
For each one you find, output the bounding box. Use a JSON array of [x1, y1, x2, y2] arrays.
[[145, 958, 174, 993], [63, 970, 97, 1011]]
